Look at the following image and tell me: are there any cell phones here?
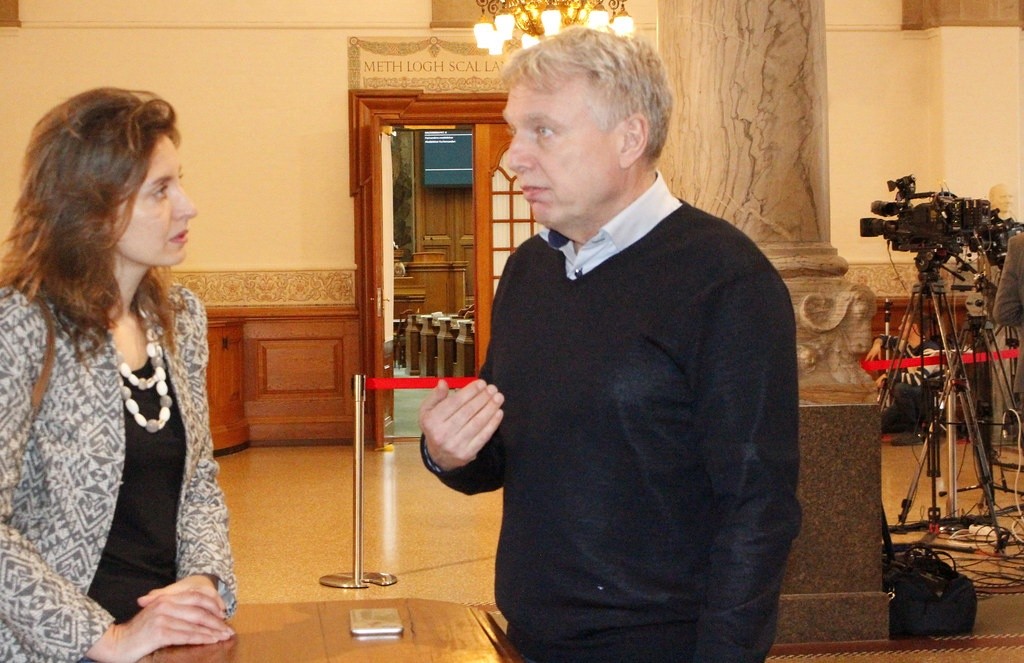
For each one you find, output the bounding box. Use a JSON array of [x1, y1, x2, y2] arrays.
[[350, 607, 404, 635]]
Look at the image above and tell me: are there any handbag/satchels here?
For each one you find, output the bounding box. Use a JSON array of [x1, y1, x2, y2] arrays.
[[883, 544, 977, 640]]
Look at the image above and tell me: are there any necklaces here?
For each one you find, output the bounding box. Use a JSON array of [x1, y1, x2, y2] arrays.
[[112, 309, 173, 433]]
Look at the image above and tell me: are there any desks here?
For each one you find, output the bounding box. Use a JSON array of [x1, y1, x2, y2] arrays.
[[139, 596, 522, 663]]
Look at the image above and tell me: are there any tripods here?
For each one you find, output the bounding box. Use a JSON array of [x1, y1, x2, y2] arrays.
[[875, 246, 1024, 557]]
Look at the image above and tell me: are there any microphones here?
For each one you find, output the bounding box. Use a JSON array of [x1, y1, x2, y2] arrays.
[[870, 202, 903, 216]]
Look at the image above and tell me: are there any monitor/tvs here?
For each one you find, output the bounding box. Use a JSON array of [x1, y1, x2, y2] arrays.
[[420, 130, 473, 188]]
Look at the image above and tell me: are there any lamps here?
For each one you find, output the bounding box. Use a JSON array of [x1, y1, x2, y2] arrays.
[[473, 0, 636, 57]]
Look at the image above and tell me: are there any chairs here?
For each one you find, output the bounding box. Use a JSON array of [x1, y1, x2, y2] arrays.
[[394, 309, 414, 369]]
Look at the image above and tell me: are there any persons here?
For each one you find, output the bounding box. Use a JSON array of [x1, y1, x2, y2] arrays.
[[992, 233, 1024, 407], [415, 25, 801, 663], [864, 313, 951, 447], [0, 88, 237, 663]]
